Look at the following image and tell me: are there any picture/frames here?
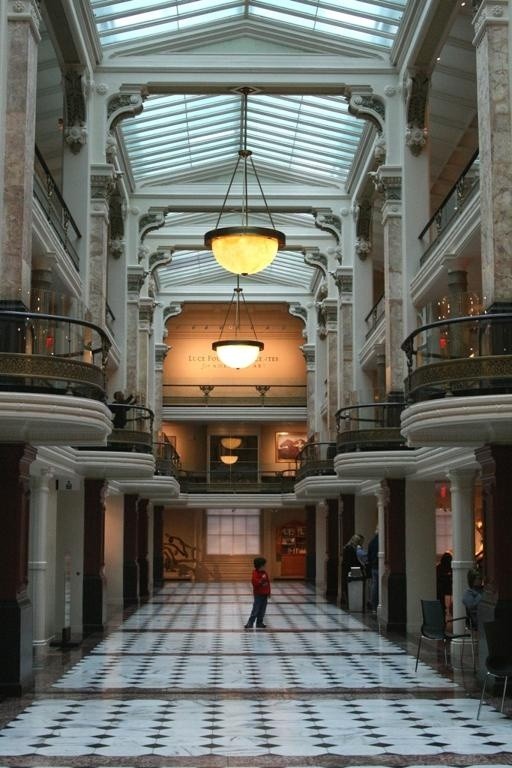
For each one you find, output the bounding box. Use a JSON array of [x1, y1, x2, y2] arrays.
[[275, 432, 308, 463]]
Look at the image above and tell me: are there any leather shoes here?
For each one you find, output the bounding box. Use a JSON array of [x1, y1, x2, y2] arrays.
[[245, 621, 267, 628]]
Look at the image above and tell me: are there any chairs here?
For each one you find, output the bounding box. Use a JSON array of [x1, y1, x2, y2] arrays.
[[415, 600, 474, 673], [477, 621, 512, 720]]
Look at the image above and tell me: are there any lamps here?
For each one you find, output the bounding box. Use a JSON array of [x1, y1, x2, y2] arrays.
[[212, 275, 264, 369], [220, 434, 243, 466], [205, 88, 285, 276]]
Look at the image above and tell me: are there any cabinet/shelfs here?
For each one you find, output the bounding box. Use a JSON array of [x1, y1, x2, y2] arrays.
[[276, 520, 306, 576]]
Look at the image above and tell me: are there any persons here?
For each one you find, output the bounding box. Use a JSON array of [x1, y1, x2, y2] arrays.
[[435, 551, 453, 607], [108, 390, 138, 429], [368, 522, 378, 619], [244, 557, 271, 628], [341, 534, 360, 609]]
